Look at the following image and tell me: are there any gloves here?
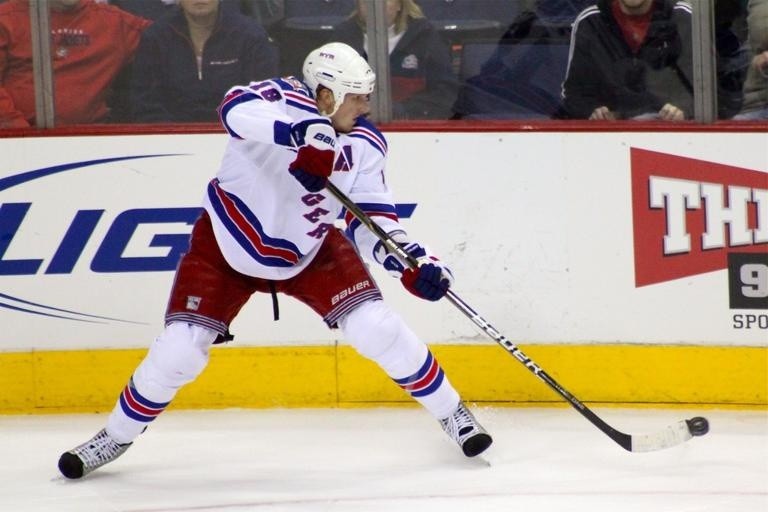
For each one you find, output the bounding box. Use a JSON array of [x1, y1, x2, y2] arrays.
[[287, 116, 342, 192], [372, 230, 455, 301]]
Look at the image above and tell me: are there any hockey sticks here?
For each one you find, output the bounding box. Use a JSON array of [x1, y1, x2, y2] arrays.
[[323, 180, 694, 452]]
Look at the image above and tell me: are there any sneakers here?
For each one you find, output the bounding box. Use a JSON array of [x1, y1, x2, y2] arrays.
[[59, 427, 134, 479], [438, 400, 492, 458]]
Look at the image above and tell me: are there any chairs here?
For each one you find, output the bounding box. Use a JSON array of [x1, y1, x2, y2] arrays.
[[282, 1, 575, 120]]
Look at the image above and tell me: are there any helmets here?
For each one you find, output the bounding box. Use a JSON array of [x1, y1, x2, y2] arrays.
[[303, 44, 376, 101]]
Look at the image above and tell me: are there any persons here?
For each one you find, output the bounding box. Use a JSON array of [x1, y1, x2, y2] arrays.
[[1, 0, 768, 132], [50, 42, 493, 481]]
